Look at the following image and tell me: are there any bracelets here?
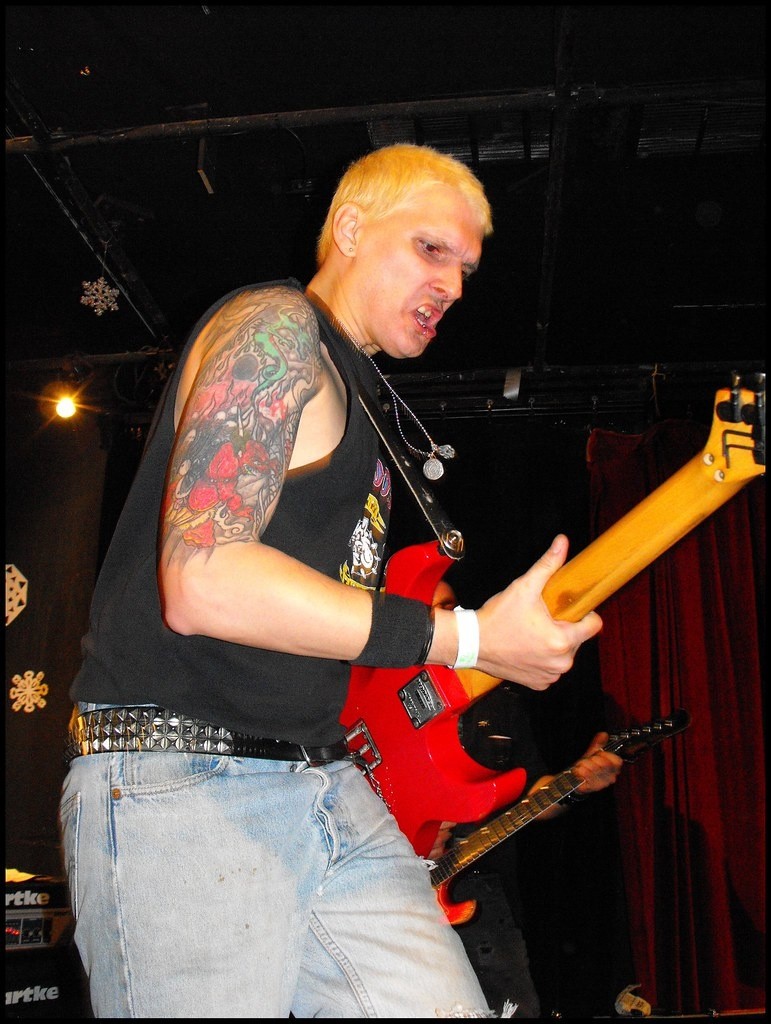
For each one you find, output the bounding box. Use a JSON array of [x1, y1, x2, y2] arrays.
[[447, 604, 480, 670], [350, 588, 433, 668]]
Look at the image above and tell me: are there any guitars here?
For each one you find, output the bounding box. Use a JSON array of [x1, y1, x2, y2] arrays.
[[428, 711, 692, 925], [340, 370, 771, 860]]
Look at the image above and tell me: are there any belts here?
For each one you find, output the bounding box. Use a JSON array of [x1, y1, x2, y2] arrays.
[[62, 706, 349, 774]]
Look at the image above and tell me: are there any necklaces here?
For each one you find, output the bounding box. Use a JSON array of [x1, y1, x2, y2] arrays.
[[318, 303, 455, 481]]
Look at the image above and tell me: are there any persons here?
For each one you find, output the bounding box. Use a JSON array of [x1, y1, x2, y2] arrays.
[[58, 145, 605, 1018], [451, 688, 624, 1017]]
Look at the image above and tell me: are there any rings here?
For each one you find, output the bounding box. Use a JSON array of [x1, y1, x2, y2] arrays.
[[605, 766, 617, 773], [598, 772, 608, 778]]
